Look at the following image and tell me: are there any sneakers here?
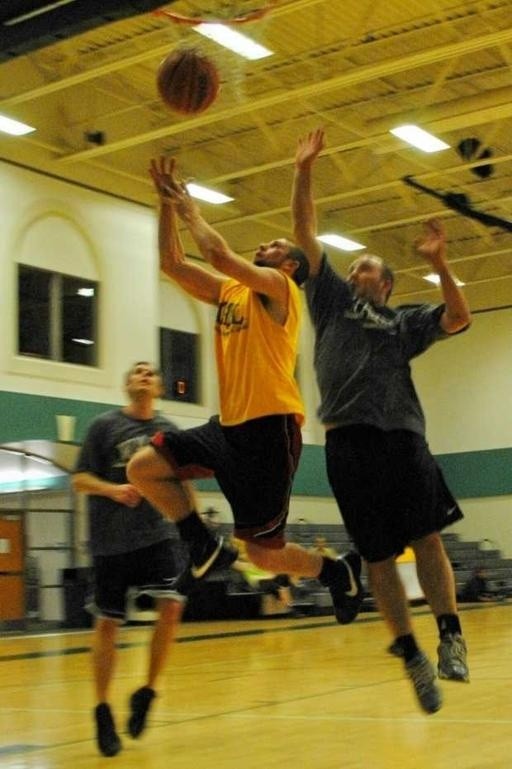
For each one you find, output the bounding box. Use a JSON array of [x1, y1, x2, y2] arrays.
[[128, 686, 156, 739], [328, 549, 363, 625], [406, 649, 443, 713], [436, 630, 469, 684], [176, 535, 239, 594], [95, 703, 121, 756]]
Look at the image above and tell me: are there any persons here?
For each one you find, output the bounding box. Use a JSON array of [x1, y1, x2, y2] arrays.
[[124, 152, 365, 628], [221, 533, 338, 612], [293, 124, 478, 717], [67, 359, 197, 759]]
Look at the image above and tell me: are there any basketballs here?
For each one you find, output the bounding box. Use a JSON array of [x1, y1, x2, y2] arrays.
[[157, 52, 218, 115]]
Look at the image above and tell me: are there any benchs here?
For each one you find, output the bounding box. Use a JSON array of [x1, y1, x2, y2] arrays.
[[195, 515, 511, 612]]
